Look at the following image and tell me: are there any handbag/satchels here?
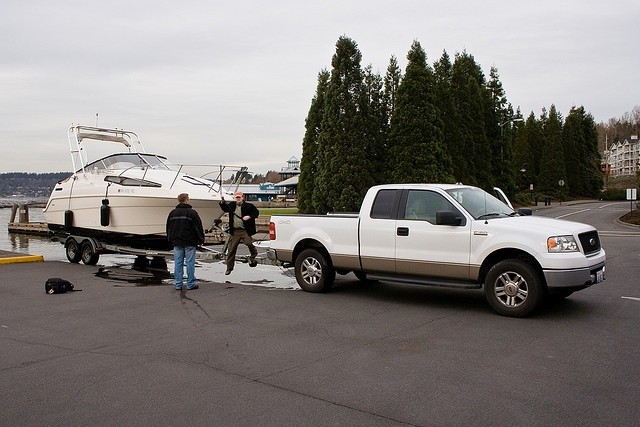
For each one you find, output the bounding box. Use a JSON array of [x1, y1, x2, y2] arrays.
[[246, 219, 257, 236]]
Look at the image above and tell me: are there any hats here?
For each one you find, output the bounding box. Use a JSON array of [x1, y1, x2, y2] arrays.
[[233, 191, 244, 198]]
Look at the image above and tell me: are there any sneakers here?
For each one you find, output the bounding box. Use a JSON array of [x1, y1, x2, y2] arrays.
[[187, 284, 199, 289], [225, 267, 233, 274], [248, 257, 254, 265], [176, 285, 182, 289]]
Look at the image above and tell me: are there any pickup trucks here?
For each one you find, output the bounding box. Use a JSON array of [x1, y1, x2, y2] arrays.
[[265, 183, 608, 319]]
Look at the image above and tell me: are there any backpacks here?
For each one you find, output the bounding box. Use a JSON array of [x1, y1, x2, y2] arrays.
[[46, 277, 82, 295]]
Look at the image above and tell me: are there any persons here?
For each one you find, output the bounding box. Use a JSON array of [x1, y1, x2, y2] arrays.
[[166, 193, 204, 290], [218, 192, 259, 275]]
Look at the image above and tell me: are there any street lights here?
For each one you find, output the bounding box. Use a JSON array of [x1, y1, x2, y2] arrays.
[[497, 118, 523, 175], [520, 163, 535, 202]]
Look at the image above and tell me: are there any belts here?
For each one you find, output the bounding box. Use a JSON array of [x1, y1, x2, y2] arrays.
[[234, 228, 245, 230]]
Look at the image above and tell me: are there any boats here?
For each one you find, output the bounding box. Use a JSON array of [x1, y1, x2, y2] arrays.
[[42, 112, 249, 244]]
